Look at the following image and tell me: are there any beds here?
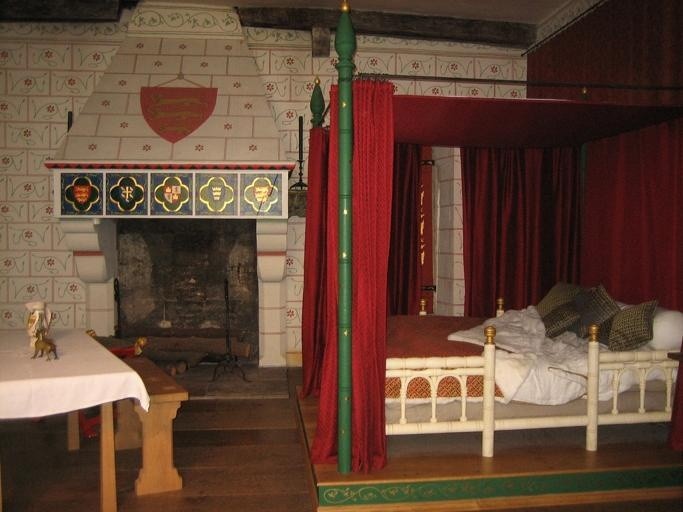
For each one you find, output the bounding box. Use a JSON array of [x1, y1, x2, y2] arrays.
[[384, 289, 683, 459]]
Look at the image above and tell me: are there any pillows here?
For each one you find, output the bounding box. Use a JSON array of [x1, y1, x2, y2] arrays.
[[531, 277, 654, 349]]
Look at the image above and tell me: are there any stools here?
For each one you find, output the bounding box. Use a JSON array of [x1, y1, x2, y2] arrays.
[[112, 355, 188, 497]]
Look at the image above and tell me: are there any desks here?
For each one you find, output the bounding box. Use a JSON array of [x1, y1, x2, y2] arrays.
[[0, 328, 138, 511]]
